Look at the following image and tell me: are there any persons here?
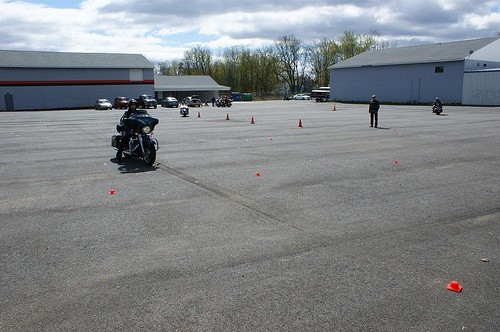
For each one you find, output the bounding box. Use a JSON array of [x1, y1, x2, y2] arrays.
[[116, 99, 139, 162], [432, 97, 441, 113], [369, 94, 379, 128], [212, 96, 215, 106]]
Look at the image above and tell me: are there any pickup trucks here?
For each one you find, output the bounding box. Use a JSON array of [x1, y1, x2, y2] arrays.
[[134, 94, 157, 108]]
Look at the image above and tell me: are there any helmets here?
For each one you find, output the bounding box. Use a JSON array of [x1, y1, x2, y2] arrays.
[[128, 99, 137, 110]]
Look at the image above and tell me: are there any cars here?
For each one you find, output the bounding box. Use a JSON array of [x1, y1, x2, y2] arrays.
[[161, 97, 178, 108], [95, 98, 112, 110], [293, 94, 310, 100], [184, 95, 202, 107]]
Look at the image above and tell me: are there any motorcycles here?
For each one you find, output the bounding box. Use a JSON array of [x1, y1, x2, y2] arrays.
[[180, 103, 190, 116], [432, 102, 443, 114], [216, 96, 233, 106], [110, 111, 160, 164]]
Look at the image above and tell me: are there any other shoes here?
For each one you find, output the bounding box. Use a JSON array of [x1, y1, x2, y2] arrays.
[[116, 154, 122, 162]]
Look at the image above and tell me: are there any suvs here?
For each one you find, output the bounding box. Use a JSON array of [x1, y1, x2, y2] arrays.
[[114, 97, 130, 108]]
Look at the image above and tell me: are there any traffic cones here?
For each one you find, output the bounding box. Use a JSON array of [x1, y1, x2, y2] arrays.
[[226, 114, 230, 120], [298, 119, 303, 127], [333, 104, 336, 111], [250, 117, 256, 124]]
